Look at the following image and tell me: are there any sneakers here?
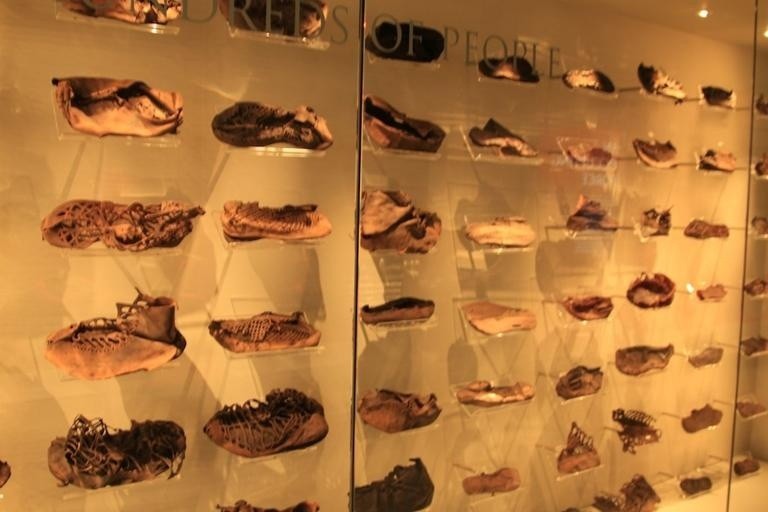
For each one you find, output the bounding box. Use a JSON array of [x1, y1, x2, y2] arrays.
[[48, 414, 186, 490], [566, 193, 768, 239], [562, 61, 768, 116], [222, 201, 332, 242], [457, 56, 537, 493], [556, 134, 768, 184], [204, 388, 328, 458], [57, 77, 184, 136], [217, 499, 320, 512], [43, 286, 186, 381], [217, 0, 327, 37], [555, 337, 768, 512], [560, 271, 768, 319], [41, 200, 205, 252], [208, 310, 321, 351], [211, 101, 333, 150], [61, 0, 183, 24]]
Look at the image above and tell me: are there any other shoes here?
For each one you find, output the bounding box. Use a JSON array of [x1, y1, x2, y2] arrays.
[[363, 95, 444, 152], [360, 189, 441, 254], [353, 457, 434, 512], [359, 388, 441, 433], [364, 23, 443, 64], [360, 296, 435, 324]]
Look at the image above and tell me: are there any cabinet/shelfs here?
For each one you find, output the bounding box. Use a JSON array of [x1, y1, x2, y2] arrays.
[[725, 1, 764, 508], [4, 0, 364, 510], [351, 0, 757, 511]]
